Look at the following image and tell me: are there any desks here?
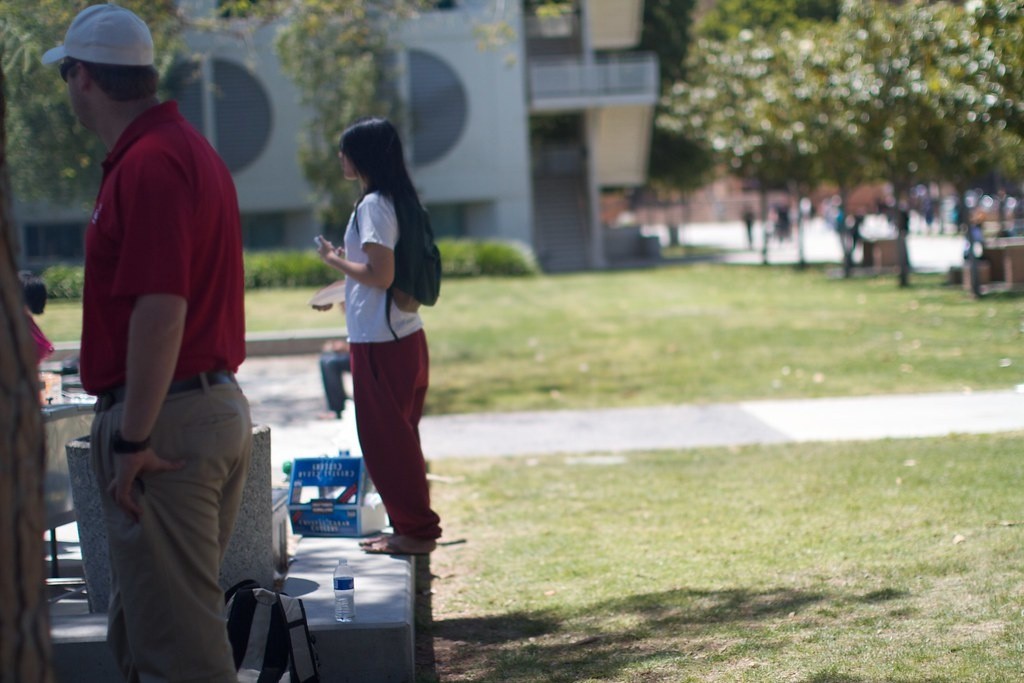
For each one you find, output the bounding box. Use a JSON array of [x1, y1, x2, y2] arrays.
[[35, 394, 99, 577]]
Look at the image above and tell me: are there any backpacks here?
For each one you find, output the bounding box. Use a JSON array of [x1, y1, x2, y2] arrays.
[[353, 190, 441, 307], [221, 578, 319, 683]]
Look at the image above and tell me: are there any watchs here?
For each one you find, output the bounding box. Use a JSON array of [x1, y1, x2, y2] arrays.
[[113, 431, 152, 455]]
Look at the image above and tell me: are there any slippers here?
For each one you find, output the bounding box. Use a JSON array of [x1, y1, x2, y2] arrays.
[[355, 533, 432, 555]]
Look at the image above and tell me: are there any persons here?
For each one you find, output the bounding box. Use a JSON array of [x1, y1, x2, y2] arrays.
[[43, 4, 252, 683], [743, 194, 1017, 262], [319, 301, 352, 419], [317, 118, 443, 552], [20, 269, 54, 403]]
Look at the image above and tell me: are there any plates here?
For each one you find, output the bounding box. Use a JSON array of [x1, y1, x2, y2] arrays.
[[308, 284, 346, 306]]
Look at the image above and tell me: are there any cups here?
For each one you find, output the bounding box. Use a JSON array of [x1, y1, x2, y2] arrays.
[[45, 374, 62, 399]]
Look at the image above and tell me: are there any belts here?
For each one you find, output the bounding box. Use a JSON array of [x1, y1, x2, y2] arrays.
[[96, 370, 235, 415]]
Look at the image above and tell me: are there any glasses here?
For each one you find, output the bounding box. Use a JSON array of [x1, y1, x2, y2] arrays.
[[56, 62, 82, 82]]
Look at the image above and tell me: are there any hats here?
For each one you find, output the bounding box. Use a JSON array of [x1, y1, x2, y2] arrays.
[[40, 3, 155, 68]]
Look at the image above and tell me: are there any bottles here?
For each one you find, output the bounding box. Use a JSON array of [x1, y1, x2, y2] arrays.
[[333, 559, 355, 622]]
